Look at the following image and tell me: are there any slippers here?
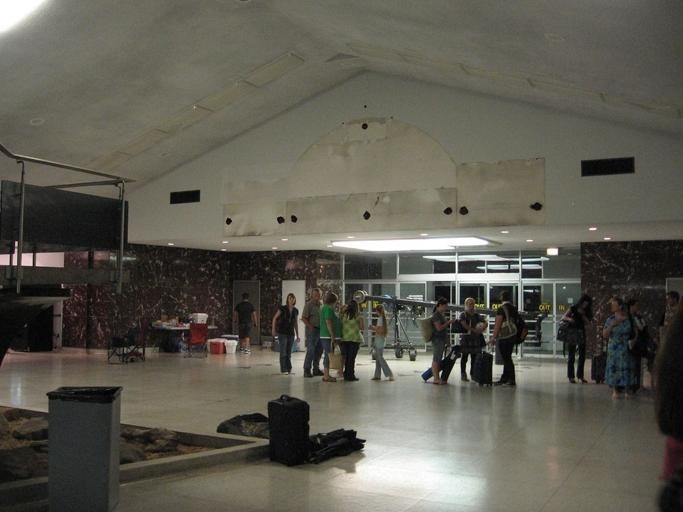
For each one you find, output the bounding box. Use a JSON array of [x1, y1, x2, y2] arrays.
[[433, 378, 445, 385]]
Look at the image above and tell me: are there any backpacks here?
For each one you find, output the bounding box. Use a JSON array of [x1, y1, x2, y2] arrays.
[[504, 304, 528, 343], [415, 310, 441, 343]]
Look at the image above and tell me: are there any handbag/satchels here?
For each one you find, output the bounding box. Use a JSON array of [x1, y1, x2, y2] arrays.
[[496, 320, 517, 339], [557, 326, 569, 341], [630, 329, 647, 354], [450, 318, 472, 336], [328, 352, 345, 370], [217, 412, 269, 440]]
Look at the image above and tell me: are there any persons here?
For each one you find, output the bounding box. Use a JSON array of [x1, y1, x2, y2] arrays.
[[603, 297, 638, 400], [459, 297, 488, 382], [342, 300, 364, 381], [301, 288, 324, 378], [319, 292, 342, 382], [430, 298, 456, 385], [652, 310, 683, 512], [489, 291, 520, 386], [337, 304, 348, 376], [562, 295, 593, 384], [627, 298, 656, 396], [663, 291, 681, 326], [367, 305, 395, 380], [234, 293, 258, 354], [272, 293, 300, 374]]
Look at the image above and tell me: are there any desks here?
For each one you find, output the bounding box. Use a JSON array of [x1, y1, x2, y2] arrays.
[[154, 325, 216, 353]]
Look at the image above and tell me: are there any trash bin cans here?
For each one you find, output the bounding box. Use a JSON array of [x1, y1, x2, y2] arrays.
[[46, 386, 123, 512]]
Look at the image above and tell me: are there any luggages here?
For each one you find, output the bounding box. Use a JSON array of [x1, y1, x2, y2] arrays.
[[470, 346, 493, 386], [269, 394, 310, 466], [591, 335, 607, 383]]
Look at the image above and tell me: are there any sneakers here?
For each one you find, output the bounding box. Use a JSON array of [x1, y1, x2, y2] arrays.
[[239, 347, 250, 354], [495, 380, 516, 385], [461, 378, 469, 382], [371, 377, 380, 380], [282, 371, 295, 375], [305, 371, 359, 383]]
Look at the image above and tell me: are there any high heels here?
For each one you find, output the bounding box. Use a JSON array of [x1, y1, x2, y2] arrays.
[[569, 377, 587, 384]]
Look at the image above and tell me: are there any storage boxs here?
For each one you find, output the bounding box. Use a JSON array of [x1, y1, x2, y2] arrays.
[[190, 312, 240, 355]]
